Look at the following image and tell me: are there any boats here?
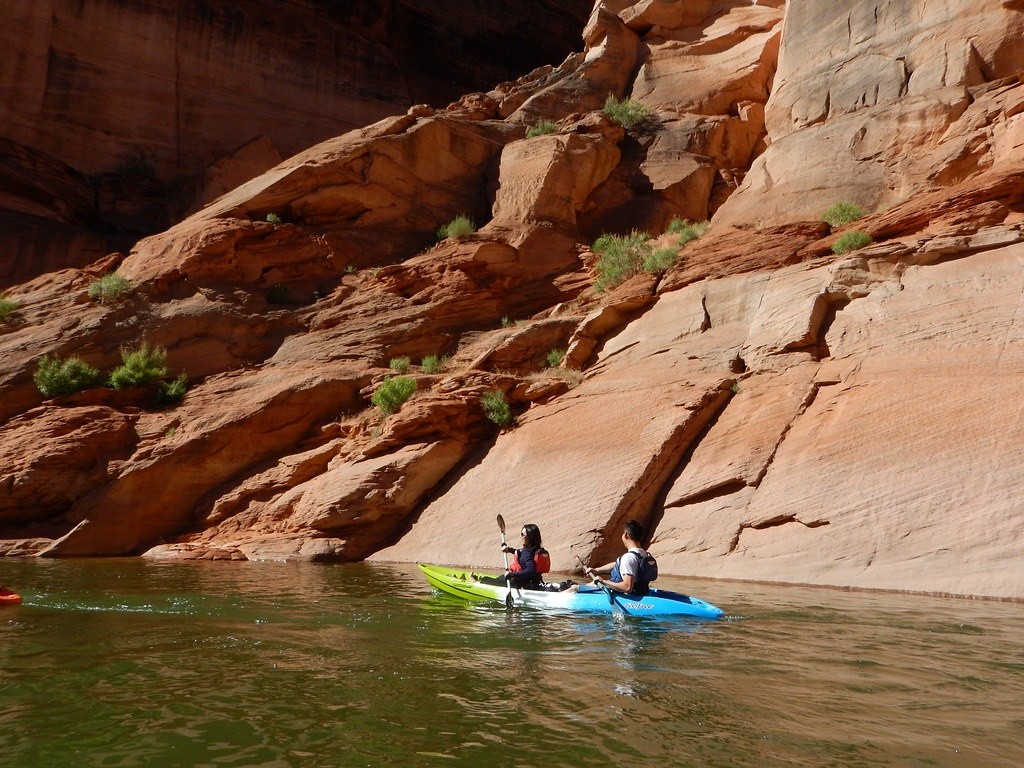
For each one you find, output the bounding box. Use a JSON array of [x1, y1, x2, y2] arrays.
[[417, 562, 726, 619]]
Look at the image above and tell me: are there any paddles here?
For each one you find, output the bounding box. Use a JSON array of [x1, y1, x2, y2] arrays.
[[576, 554, 616, 606], [496, 513, 515, 609]]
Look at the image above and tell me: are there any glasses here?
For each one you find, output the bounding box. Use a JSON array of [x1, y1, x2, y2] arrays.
[[521, 533, 527, 537], [621, 531, 626, 536]]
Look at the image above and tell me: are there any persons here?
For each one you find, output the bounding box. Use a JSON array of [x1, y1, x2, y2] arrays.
[[468, 524, 542, 590], [559, 520, 658, 594]]
